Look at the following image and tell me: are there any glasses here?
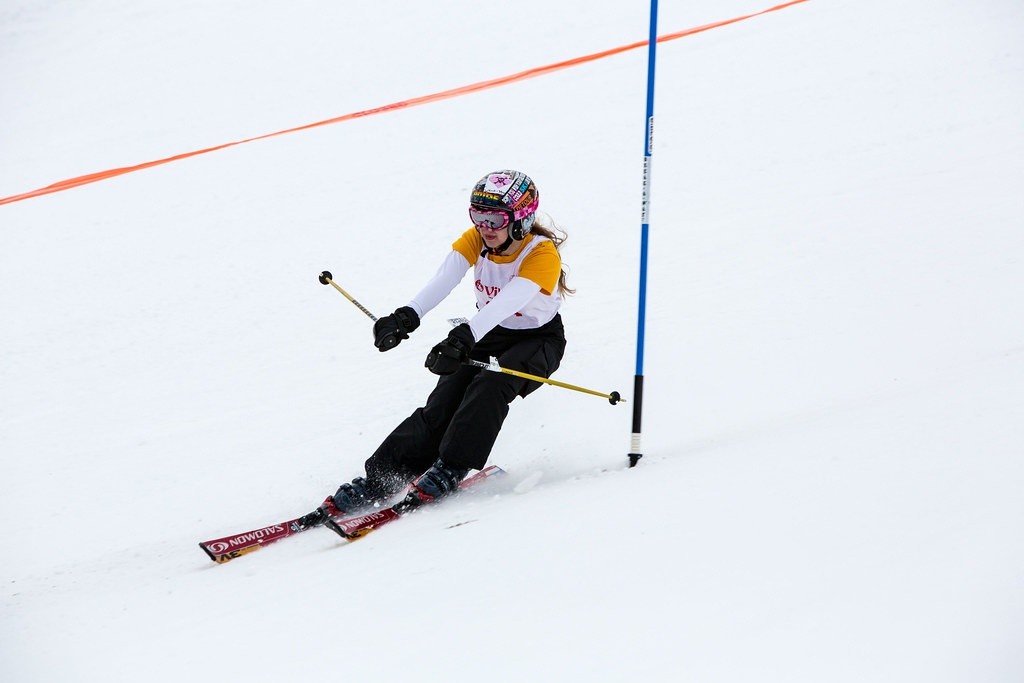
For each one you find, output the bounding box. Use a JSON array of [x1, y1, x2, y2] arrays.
[[469, 192, 539, 232]]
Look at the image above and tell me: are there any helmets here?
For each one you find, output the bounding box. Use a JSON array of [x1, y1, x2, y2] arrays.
[[470, 169, 539, 241]]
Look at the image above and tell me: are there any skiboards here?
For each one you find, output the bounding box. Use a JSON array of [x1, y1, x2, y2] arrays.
[[200, 465, 508, 568]]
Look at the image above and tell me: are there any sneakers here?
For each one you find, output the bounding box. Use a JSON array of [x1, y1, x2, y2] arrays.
[[408, 459, 472, 502], [333, 476, 394, 514]]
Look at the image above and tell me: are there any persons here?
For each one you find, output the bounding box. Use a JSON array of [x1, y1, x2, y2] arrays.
[[331, 170, 575, 516]]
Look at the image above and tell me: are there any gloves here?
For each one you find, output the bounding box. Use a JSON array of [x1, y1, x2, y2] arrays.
[[425, 323, 476, 376], [373, 306, 420, 352]]
